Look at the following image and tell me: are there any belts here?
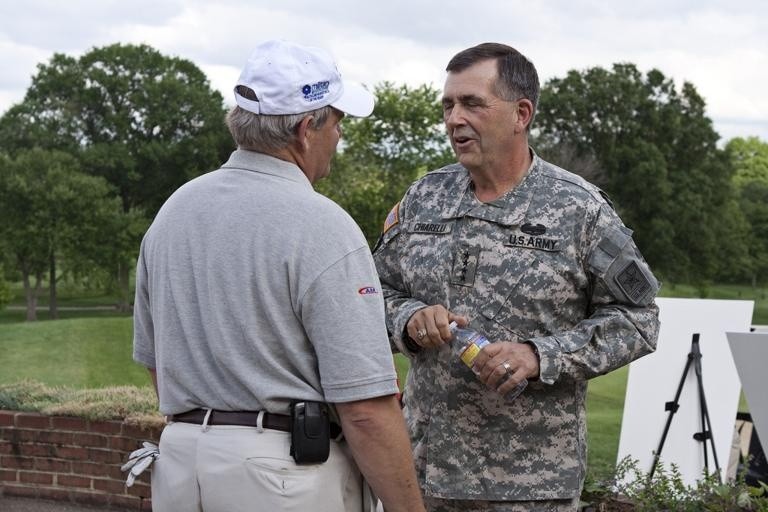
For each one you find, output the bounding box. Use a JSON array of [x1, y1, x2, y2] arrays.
[[173, 409, 340, 440]]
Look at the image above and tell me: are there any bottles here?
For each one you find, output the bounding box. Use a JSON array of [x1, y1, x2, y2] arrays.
[[443, 320, 530, 403]]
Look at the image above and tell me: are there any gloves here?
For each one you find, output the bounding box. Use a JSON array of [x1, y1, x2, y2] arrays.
[[121, 442, 158, 488]]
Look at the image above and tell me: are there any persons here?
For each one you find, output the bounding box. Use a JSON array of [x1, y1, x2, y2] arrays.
[[132, 37, 428, 512], [372, 42, 659, 511]]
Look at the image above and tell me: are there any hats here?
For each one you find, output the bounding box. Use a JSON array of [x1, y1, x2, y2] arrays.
[[233, 40, 376, 119]]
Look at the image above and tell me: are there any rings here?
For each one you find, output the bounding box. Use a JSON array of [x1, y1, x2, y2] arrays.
[[416, 328, 428, 340], [502, 361, 513, 374]]
